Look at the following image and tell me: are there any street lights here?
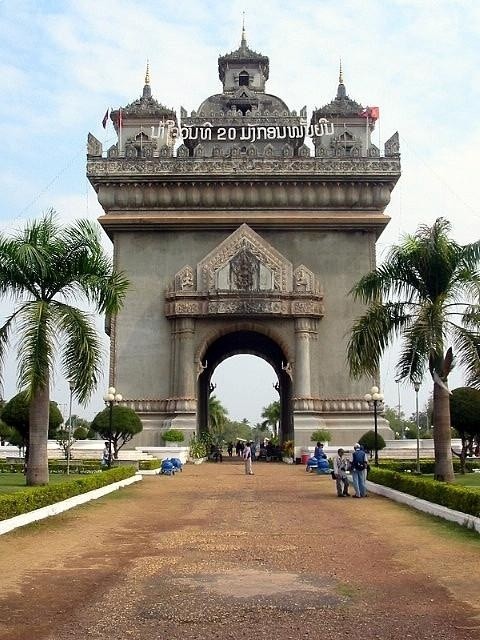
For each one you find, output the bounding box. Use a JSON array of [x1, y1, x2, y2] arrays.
[[395, 379, 402, 420], [412, 381, 422, 473], [103, 387, 123, 469], [67, 380, 77, 476], [364, 386, 384, 467]]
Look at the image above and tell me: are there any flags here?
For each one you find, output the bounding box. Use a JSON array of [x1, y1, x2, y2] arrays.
[[102, 110, 108, 129], [371, 108, 379, 119], [362, 109, 367, 120], [118, 111, 124, 128]]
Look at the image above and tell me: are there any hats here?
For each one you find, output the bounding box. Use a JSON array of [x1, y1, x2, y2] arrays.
[[355, 443, 360, 447]]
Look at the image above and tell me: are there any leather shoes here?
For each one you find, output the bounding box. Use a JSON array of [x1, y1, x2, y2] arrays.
[[338, 491, 360, 498]]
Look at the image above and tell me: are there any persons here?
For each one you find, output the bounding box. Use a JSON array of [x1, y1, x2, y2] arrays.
[[227, 440, 257, 475], [103, 441, 113, 465], [351, 442, 369, 498], [315, 442, 327, 459], [334, 448, 351, 498]]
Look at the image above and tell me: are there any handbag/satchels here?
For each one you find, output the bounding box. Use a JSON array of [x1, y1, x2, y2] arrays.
[[244, 454, 250, 458], [331, 471, 337, 479]]
